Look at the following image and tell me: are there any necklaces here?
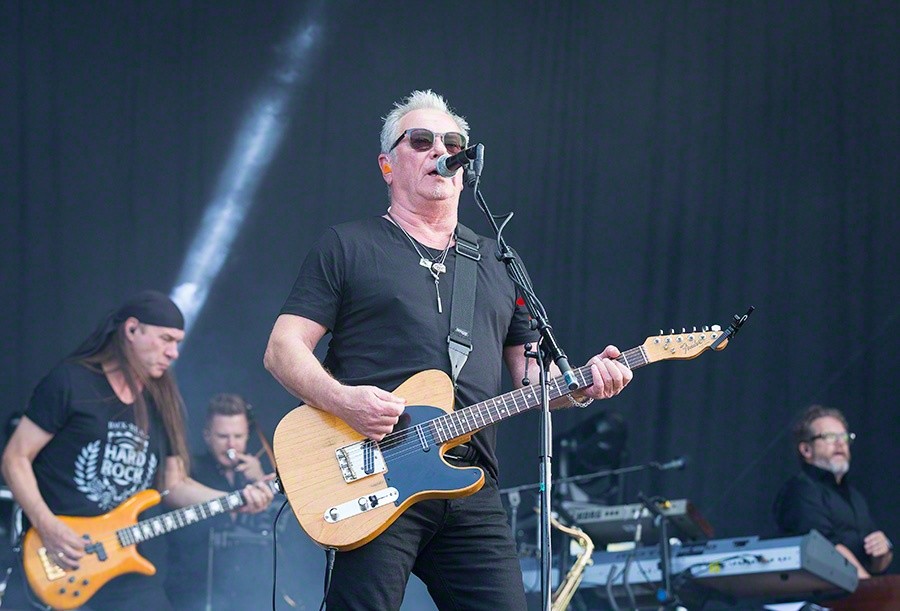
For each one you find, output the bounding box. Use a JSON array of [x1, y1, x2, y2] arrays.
[[387, 206, 454, 313]]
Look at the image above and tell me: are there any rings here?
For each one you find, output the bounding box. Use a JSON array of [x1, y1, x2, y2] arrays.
[[58, 552, 64, 559]]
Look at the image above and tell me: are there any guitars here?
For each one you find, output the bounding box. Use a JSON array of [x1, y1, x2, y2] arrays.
[[272, 304, 756, 553], [22, 476, 283, 611]]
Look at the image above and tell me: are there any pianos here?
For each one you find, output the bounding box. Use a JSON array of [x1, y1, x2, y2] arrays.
[[518, 528, 858, 611]]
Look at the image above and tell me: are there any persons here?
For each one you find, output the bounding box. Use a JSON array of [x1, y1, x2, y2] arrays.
[[773, 404, 894, 579], [262, 88, 634, 611], [0, 290, 277, 611], [162, 393, 264, 611]]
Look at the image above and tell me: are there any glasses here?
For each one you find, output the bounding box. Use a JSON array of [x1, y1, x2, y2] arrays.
[[389, 128, 466, 152], [803, 430, 856, 443]]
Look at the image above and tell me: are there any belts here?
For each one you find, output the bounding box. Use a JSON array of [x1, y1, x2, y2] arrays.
[[444, 445, 480, 465]]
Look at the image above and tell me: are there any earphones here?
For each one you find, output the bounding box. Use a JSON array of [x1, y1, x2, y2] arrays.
[[383, 165, 392, 173], [130, 328, 134, 333]]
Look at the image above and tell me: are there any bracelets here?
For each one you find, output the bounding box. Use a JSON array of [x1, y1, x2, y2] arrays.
[[888, 538, 893, 551], [565, 394, 595, 408]]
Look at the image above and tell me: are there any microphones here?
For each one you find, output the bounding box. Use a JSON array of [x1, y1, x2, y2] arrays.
[[436, 143, 485, 178], [661, 458, 686, 472]]
